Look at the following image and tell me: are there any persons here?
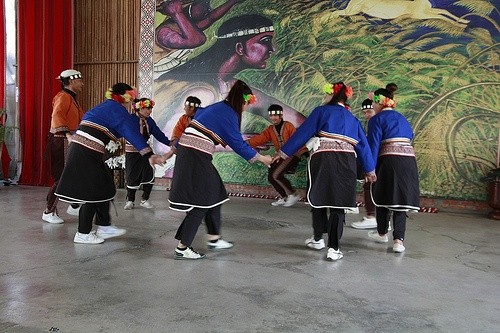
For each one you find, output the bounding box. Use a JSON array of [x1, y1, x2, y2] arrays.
[[160, 79, 273, 260], [367, 83, 419, 253], [271, 82, 377, 261], [245, 104, 307, 207], [41, 69, 85, 224], [54, 82, 166, 244], [124, 98, 174, 209], [171, 96, 201, 143], [344, 98, 391, 231]]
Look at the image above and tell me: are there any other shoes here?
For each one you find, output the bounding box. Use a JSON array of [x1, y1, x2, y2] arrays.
[[368, 230, 388, 243], [350, 216, 377, 228], [304, 235, 326, 250], [326, 248, 343, 261], [124, 201, 135, 209], [67, 205, 80, 215], [140, 199, 154, 208], [174, 246, 206, 259], [73, 230, 105, 243], [208, 236, 234, 249], [96, 225, 127, 239], [284, 194, 301, 207], [344, 207, 359, 214], [393, 243, 405, 253], [272, 196, 287, 206], [388, 221, 391, 231], [42, 210, 64, 223]]
